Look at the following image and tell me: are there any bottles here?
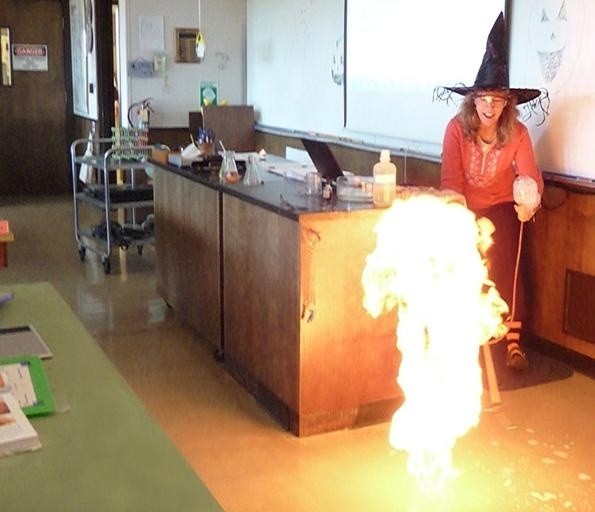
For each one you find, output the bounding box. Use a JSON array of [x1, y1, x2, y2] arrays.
[[371, 150, 397, 208], [219, 151, 261, 187], [323, 177, 333, 201]]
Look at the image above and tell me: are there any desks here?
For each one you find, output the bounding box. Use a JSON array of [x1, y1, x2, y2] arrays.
[[0, 282, 223, 512]]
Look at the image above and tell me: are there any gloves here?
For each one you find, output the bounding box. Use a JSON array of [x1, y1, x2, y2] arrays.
[[514, 204, 541, 222]]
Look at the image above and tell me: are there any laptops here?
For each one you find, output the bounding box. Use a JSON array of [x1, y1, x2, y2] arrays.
[[300, 137, 374, 185]]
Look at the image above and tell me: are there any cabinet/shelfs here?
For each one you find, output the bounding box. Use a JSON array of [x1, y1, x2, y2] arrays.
[[71, 136, 153, 258]]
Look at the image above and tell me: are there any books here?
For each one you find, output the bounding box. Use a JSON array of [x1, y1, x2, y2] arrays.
[[0, 397, 43, 454], [438, 11, 547, 373]]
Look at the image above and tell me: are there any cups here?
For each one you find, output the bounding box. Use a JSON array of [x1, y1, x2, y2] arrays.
[[305, 172, 321, 196]]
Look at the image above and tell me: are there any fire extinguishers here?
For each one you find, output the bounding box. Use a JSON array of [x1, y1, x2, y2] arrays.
[[128, 97, 154, 147]]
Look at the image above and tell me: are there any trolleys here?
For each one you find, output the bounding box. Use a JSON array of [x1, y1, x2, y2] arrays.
[[70, 138, 170, 274]]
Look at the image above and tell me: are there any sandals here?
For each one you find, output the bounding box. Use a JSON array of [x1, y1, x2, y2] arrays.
[[506, 346, 526, 370]]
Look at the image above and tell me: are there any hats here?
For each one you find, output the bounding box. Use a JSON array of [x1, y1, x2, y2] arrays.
[[444, 11, 541, 105]]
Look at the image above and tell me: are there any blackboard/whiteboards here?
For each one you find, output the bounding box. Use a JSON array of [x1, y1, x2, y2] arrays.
[[246, 0, 595, 188]]
